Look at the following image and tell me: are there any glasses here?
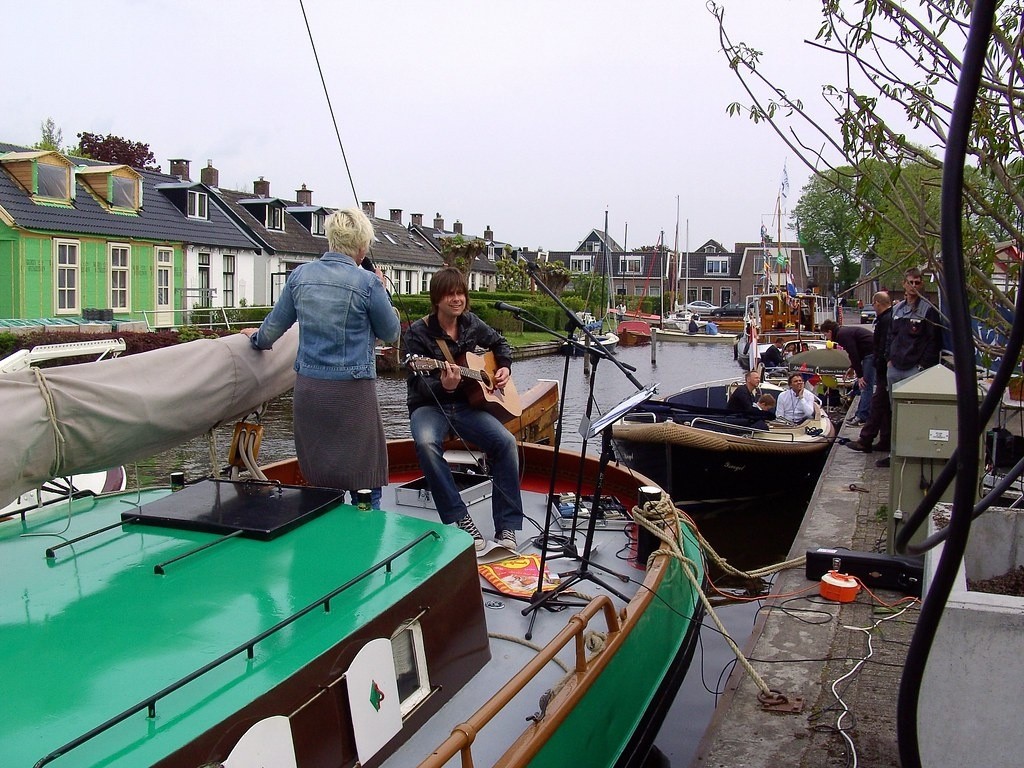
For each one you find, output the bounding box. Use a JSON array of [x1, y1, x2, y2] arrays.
[[904, 294, 907, 296], [908, 281, 921, 285]]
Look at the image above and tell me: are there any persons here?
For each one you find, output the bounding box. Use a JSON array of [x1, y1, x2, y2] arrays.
[[402, 267, 524, 552], [615, 301, 626, 323], [706, 319, 718, 335], [726, 268, 944, 467], [688, 319, 699, 336], [240, 209, 401, 512]]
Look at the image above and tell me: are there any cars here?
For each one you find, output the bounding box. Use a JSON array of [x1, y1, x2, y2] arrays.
[[712, 301, 746, 316], [677, 300, 721, 316]]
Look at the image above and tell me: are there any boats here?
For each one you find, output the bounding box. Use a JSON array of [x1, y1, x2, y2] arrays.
[[600, 374, 838, 543], [560, 194, 858, 406], [1, 294, 716, 768]]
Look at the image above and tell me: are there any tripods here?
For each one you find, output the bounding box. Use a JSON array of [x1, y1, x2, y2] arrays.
[[482, 263, 653, 642]]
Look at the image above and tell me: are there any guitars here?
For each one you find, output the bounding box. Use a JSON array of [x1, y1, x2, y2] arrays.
[[401, 349, 523, 425]]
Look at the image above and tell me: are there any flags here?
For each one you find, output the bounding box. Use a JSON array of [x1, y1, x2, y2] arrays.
[[797, 231, 808, 245], [776, 252, 786, 267], [781, 158, 791, 198], [786, 271, 798, 298]]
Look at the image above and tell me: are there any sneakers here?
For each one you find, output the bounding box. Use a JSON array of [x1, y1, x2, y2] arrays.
[[875, 457, 890, 467], [456, 512, 486, 550], [494, 529, 516, 550], [845, 416, 866, 428], [846, 438, 872, 453], [872, 441, 891, 452]]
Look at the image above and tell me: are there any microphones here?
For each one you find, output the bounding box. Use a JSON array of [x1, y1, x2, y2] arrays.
[[494, 301, 526, 315], [361, 257, 376, 275]]
